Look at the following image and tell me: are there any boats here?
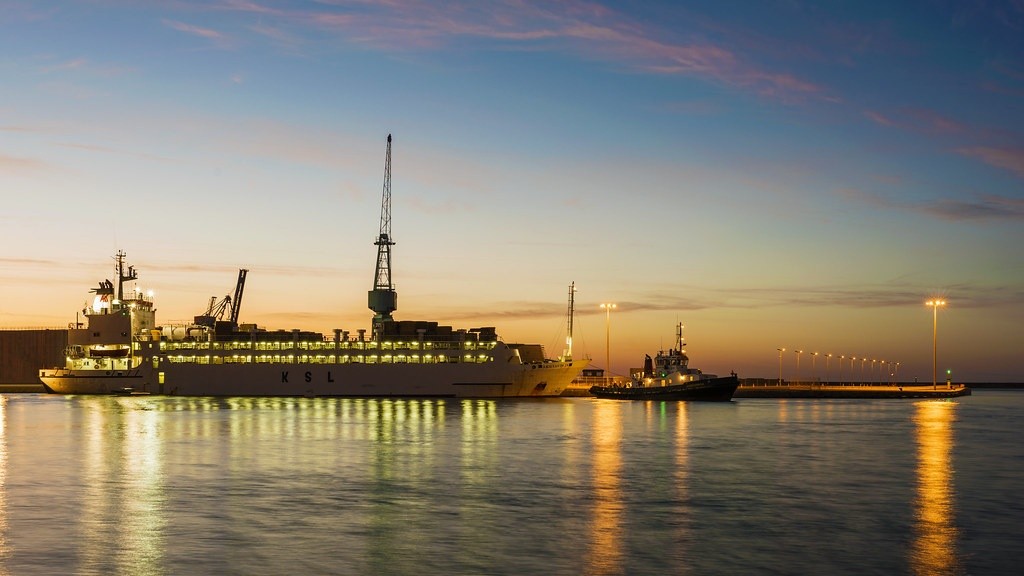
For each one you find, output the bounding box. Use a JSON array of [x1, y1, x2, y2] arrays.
[[588, 322, 741, 403]]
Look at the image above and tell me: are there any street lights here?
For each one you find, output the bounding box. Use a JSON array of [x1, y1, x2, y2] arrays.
[[824, 353, 832, 386], [849, 357, 856, 386], [597, 303, 616, 386], [837, 355, 845, 387], [878, 360, 884, 386], [860, 358, 867, 386], [887, 360, 892, 387], [869, 359, 876, 386], [794, 350, 803, 387], [776, 348, 785, 387], [810, 352, 818, 385], [894, 362, 899, 385], [925, 300, 946, 389]]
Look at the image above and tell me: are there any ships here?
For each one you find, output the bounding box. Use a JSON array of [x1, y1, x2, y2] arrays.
[[38, 132, 592, 402]]
